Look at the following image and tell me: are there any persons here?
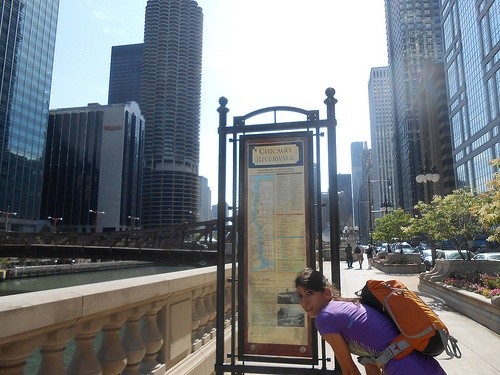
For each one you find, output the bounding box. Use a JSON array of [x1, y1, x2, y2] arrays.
[[372, 244, 376, 255], [354, 243, 364, 270], [294, 271, 447, 375], [365, 244, 373, 270], [345, 244, 354, 268]]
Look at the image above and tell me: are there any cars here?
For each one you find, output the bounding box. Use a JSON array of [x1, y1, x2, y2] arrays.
[[380, 242, 500, 271]]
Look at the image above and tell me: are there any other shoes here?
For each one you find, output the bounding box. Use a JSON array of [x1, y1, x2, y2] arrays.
[[350, 266, 353, 268]]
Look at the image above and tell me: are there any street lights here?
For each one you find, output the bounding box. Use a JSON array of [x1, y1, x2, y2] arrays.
[[48, 216, 63, 233], [0, 211, 17, 231], [343, 225, 359, 237], [128, 216, 139, 230], [189, 210, 200, 243], [415, 150, 440, 270], [361, 140, 391, 246], [380, 197, 394, 254], [89, 209, 105, 233]]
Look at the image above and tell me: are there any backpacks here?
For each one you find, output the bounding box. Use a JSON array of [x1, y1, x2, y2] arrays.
[[354, 246, 360, 254], [334, 279, 461, 369]]
